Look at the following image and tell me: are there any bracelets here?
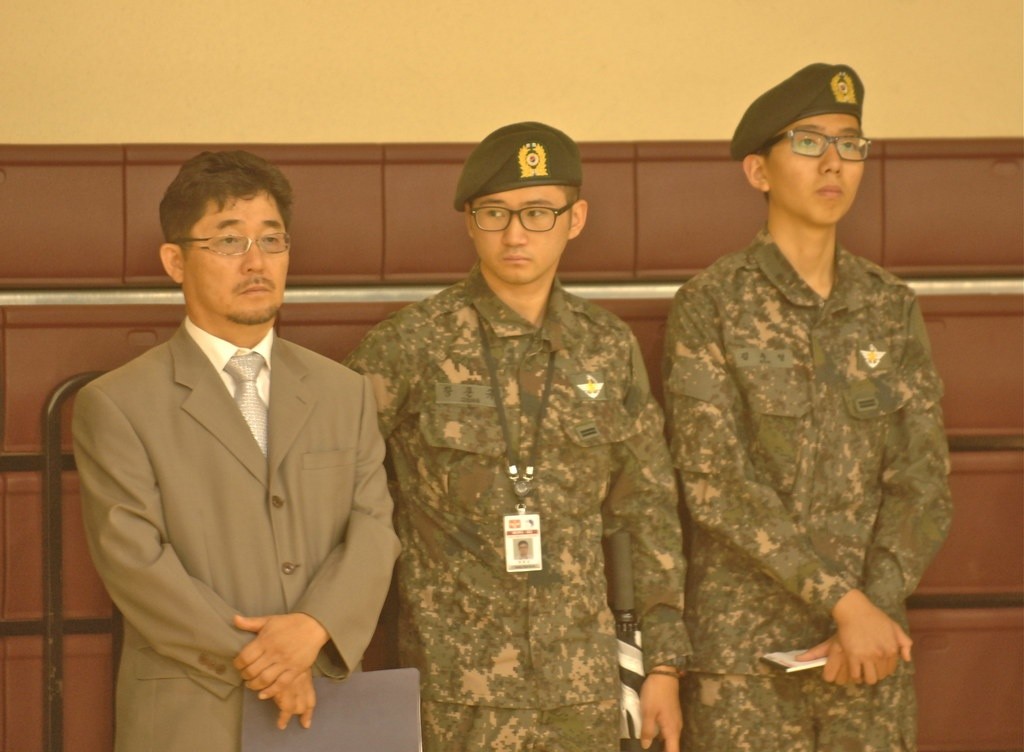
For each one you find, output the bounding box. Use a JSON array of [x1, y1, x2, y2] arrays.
[[646, 662, 688, 679]]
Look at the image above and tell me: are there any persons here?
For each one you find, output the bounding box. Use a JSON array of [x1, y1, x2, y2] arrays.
[[72, 150, 403, 752], [660, 61, 955, 752], [518, 541, 529, 559], [337, 123, 694, 752]]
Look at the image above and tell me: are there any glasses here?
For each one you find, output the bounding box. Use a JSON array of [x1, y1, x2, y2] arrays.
[[170, 232, 290, 256], [753, 130, 872, 161], [468, 201, 574, 232]]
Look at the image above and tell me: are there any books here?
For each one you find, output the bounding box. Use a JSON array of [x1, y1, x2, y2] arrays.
[[760, 648, 828, 674], [240, 667, 423, 752]]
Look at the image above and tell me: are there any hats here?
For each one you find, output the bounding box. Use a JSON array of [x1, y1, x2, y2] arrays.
[[454, 122, 582, 212], [731, 64, 865, 161]]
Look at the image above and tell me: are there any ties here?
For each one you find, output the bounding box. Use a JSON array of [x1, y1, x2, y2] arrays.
[[223, 352, 270, 458]]
